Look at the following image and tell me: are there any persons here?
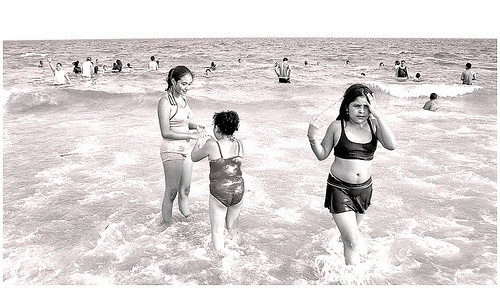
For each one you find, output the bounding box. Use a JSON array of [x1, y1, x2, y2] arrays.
[[189, 110, 246, 250], [147, 56, 157, 71], [158, 66, 206, 223], [47, 59, 70, 85], [274, 58, 291, 83], [462, 63, 476, 85], [423, 92, 437, 112], [396, 60, 408, 78], [82, 57, 94, 78], [37, 60, 424, 84], [308, 84, 398, 266]]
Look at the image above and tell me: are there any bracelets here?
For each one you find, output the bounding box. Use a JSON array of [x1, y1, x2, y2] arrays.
[[309, 139, 314, 141]]
